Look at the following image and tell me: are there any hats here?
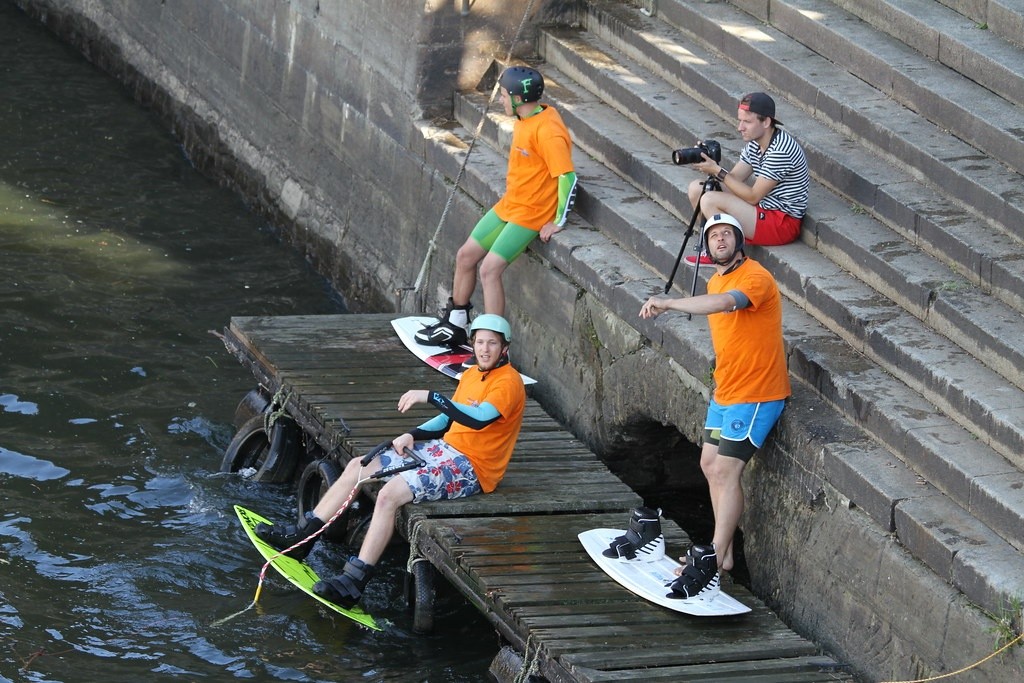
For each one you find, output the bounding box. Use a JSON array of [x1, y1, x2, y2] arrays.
[[739, 93, 785, 126]]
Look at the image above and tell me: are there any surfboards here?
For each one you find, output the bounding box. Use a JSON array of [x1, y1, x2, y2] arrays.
[[233, 504, 382, 632], [388, 316, 540, 390], [576, 528, 752, 618]]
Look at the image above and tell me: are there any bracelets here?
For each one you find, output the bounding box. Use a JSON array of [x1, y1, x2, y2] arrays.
[[716, 168, 729, 181]]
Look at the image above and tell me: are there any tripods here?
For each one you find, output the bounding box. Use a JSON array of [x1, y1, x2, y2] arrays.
[[665, 174, 724, 320]]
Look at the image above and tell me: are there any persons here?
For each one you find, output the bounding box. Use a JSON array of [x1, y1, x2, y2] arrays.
[[413, 65, 577, 368], [682, 91, 810, 267], [255, 314, 527, 609], [637, 213, 790, 575]]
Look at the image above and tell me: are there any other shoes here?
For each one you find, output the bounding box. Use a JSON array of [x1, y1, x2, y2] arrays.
[[602, 507, 663, 559], [666, 544, 717, 599]]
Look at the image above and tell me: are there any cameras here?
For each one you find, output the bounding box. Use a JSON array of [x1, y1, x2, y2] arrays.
[[672, 140, 721, 166]]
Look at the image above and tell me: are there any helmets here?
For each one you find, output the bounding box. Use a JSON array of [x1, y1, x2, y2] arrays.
[[704, 213, 745, 253], [499, 66, 544, 102], [469, 313, 511, 342]]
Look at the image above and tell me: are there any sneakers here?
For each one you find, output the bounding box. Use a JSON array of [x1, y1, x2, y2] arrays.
[[312, 556, 376, 610], [254, 511, 325, 560], [684, 249, 717, 267], [462, 356, 477, 368], [414, 297, 473, 349]]
